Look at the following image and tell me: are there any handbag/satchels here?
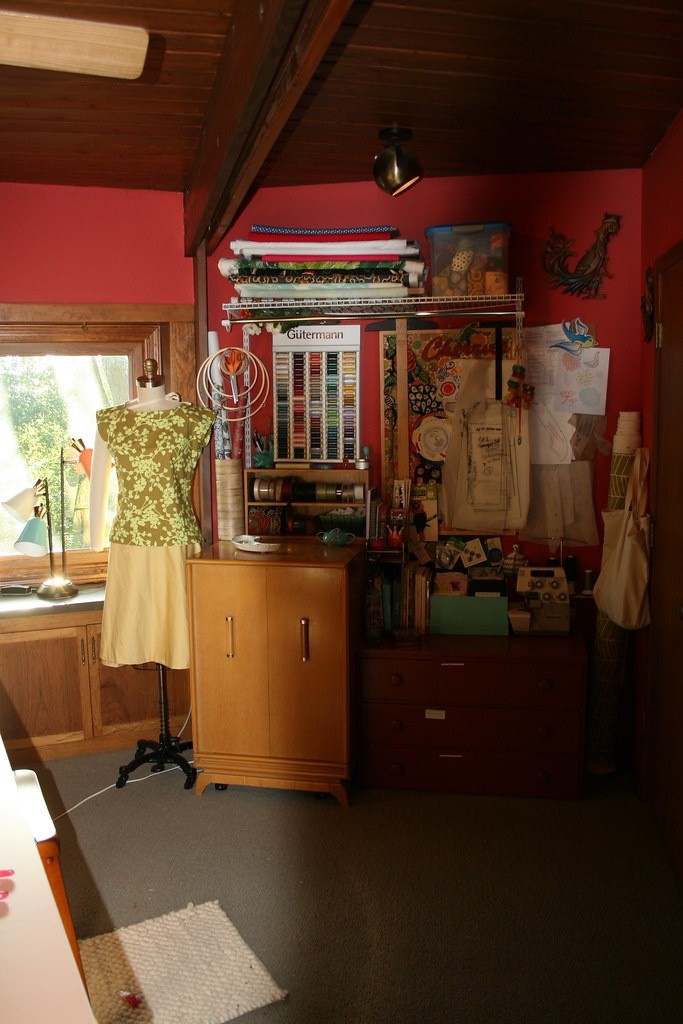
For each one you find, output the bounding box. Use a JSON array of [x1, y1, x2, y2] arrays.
[[593, 448, 651, 630]]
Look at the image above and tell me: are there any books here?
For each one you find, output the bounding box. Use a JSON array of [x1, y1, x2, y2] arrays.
[[401, 559, 433, 634]]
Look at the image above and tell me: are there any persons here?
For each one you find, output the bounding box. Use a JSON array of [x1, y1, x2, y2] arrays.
[[91, 359, 216, 672]]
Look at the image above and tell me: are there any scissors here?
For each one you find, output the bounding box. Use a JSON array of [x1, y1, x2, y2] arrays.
[[222, 348, 246, 404]]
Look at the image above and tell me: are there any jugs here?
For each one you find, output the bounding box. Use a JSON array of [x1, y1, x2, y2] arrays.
[[316, 528, 355, 546]]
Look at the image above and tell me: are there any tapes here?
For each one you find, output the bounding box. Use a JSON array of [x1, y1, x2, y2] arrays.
[[249, 476, 364, 504], [287, 516, 307, 532]]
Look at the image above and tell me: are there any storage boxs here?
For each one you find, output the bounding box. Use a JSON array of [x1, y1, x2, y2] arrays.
[[424, 221, 512, 308]]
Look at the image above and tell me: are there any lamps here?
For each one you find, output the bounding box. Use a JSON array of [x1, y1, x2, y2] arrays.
[[372, 127, 422, 196], [2, 438, 114, 600]]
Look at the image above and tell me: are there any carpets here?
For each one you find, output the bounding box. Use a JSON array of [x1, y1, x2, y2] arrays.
[[77, 901, 288, 1024]]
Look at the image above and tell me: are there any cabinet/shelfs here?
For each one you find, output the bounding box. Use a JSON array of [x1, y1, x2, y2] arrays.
[[244, 469, 370, 547], [184, 541, 366, 809], [362, 632, 589, 800], [0, 610, 192, 766]]
